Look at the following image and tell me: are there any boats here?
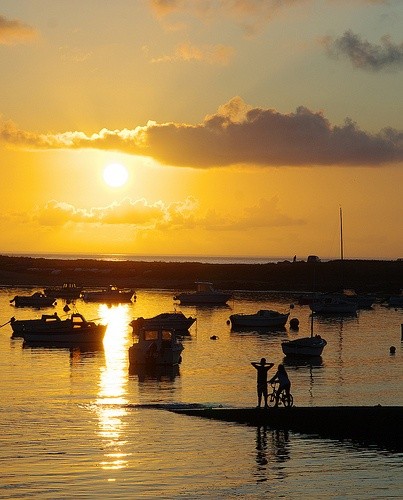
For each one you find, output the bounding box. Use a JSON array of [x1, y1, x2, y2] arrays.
[[10, 313, 108, 341], [130, 311, 197, 332], [298, 291, 376, 315], [44, 280, 82, 299], [229, 310, 291, 328], [10, 292, 57, 307], [80, 284, 135, 301], [173, 280, 234, 305], [280, 334, 327, 357], [128, 327, 185, 365]]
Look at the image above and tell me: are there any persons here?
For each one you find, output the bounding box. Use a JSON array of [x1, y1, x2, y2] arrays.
[[251, 358, 274, 408], [268, 364, 291, 407]]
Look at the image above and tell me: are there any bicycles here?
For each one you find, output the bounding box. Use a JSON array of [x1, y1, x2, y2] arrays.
[[265, 380, 294, 407]]
